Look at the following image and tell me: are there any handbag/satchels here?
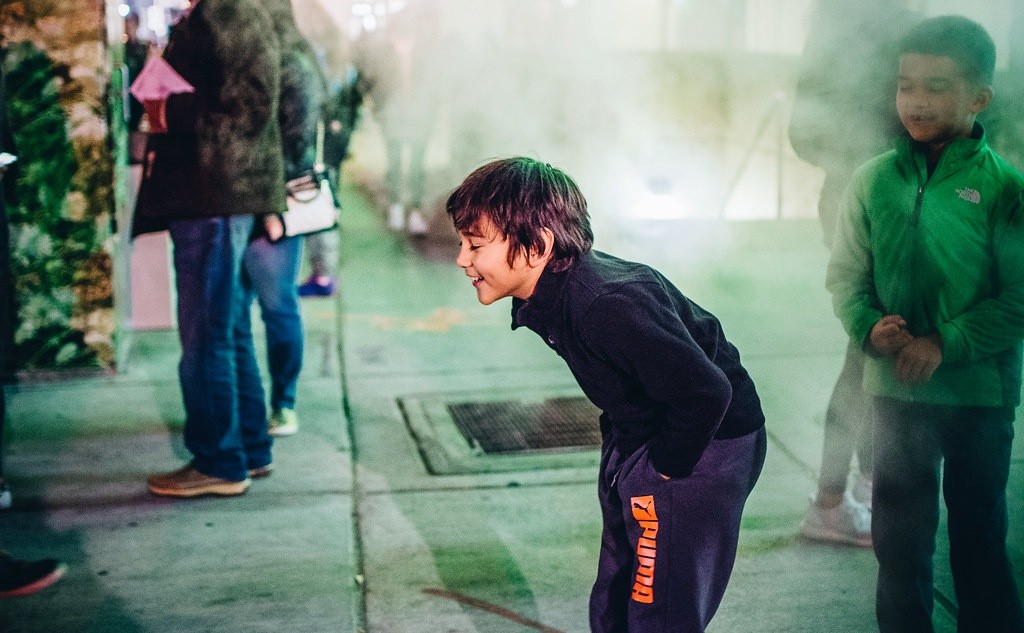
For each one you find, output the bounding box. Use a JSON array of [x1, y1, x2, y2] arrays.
[[263, 164, 342, 245]]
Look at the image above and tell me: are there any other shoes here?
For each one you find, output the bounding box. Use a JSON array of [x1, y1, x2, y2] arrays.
[[801, 491, 873, 547], [387, 206, 406, 230], [249, 462, 274, 478], [0, 550, 68, 599], [267, 406, 298, 436], [0, 480, 45, 515], [149, 464, 250, 495], [298, 275, 334, 295], [845, 489, 873, 510], [409, 212, 428, 235]]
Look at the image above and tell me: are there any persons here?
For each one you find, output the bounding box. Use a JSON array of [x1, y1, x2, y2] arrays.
[[0, 0, 365, 598], [788, 2, 1024, 633], [367, 1, 440, 237], [446, 158, 768, 633]]
